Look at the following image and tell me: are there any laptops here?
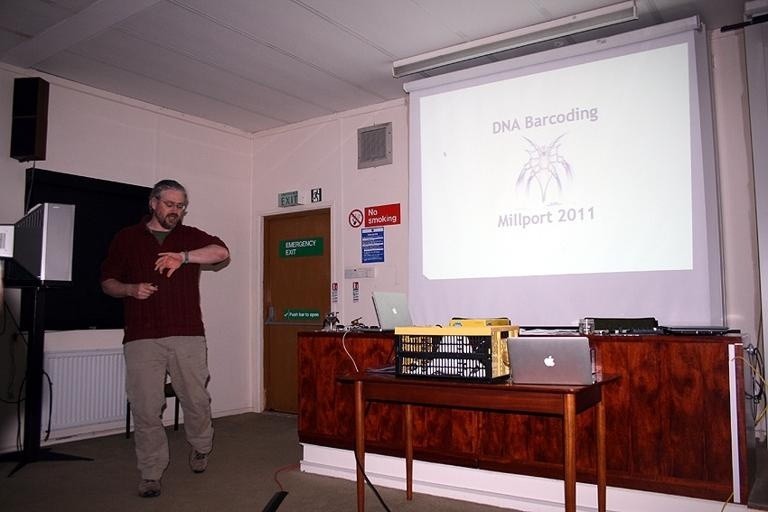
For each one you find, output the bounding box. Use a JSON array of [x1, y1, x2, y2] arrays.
[[507, 337, 597, 386], [659, 325, 729, 335], [357, 292, 413, 332]]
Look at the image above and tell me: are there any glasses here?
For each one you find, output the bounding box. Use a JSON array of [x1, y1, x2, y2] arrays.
[[160, 202, 186, 211]]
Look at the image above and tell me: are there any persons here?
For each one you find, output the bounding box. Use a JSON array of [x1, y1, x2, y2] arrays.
[[97, 179, 230, 497]]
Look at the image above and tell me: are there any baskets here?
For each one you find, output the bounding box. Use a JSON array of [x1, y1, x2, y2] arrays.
[[391, 333, 508, 383]]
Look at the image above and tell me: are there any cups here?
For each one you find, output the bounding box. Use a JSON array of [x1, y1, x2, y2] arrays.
[[578, 319, 596, 336]]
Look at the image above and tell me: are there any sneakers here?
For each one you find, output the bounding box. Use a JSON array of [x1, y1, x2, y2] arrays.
[[187, 445, 209, 473], [138, 479, 161, 497]]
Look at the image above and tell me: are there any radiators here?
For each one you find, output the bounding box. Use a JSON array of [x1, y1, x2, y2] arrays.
[[39, 348, 129, 432]]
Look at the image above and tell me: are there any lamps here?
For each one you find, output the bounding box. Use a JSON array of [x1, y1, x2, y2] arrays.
[[390, 2, 641, 83]]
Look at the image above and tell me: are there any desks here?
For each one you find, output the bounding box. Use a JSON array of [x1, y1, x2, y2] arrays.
[[335, 364, 620, 512]]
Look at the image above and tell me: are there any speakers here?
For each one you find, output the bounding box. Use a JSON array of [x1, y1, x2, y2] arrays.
[[10, 77, 49, 163]]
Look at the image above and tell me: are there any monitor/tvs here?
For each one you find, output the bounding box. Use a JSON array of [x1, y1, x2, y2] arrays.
[[0, 203, 76, 282], [19, 167, 153, 330]]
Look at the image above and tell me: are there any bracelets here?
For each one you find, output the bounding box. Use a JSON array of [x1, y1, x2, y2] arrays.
[[184, 250, 189, 263]]
